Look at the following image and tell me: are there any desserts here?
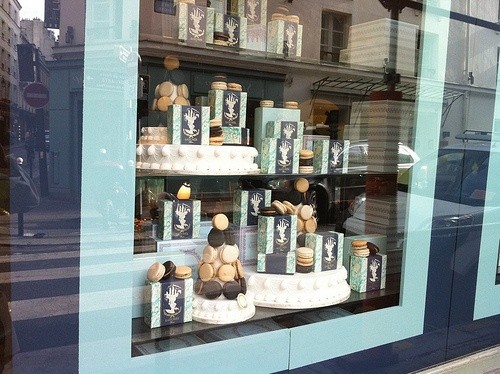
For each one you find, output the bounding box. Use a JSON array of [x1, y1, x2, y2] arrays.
[[150, 1, 301, 145], [257, 150, 380, 274], [146, 214, 247, 299]]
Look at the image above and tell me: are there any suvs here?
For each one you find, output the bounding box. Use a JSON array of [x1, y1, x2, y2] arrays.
[[344, 128, 499, 290], [238, 136, 421, 226]]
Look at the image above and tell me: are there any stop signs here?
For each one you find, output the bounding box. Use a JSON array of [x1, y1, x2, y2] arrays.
[[23, 83, 50, 110]]
